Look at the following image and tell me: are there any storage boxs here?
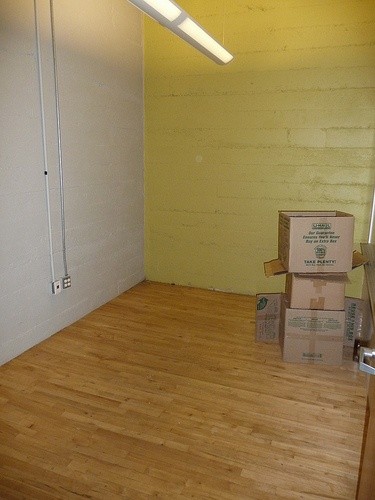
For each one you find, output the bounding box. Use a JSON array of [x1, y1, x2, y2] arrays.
[[278, 206, 353, 273], [261, 249, 368, 311], [255, 293, 370, 365]]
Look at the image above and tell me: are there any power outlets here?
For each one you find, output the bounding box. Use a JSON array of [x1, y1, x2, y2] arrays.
[[63, 276, 71, 289], [52, 280, 61, 294]]
